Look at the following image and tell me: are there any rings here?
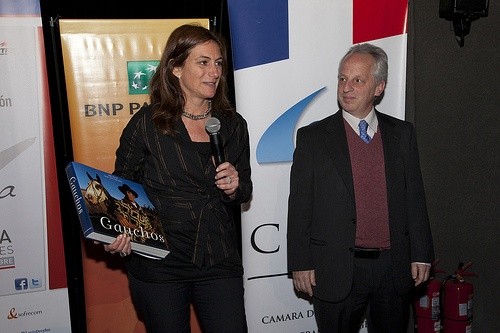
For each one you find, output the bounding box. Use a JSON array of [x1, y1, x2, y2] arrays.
[[227, 176, 232, 184], [121, 252, 127, 257]]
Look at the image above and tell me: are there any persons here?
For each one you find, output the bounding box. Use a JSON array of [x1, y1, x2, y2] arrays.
[[93, 24, 253, 333], [288, 42, 436, 333]]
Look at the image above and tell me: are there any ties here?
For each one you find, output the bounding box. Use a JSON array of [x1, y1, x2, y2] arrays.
[[359, 120, 371, 144]]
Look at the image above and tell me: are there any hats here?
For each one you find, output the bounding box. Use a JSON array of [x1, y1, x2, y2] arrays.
[[118, 184, 138, 198]]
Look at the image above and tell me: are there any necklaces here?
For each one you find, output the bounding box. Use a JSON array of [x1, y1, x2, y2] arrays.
[[183, 100, 212, 119]]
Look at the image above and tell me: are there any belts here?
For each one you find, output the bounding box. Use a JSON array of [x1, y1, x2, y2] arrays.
[[353, 248, 392, 259]]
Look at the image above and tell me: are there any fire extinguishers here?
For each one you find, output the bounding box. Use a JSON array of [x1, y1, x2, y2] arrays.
[[411, 256, 445, 333], [439, 258, 478, 333]]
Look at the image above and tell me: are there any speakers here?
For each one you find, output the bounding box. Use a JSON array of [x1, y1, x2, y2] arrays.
[[439, 0, 488, 21]]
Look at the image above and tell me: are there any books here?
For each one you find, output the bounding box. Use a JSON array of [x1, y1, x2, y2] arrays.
[[65, 162, 170, 261]]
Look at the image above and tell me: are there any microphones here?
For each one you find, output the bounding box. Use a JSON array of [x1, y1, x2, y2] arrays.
[[205, 117, 226, 179]]
